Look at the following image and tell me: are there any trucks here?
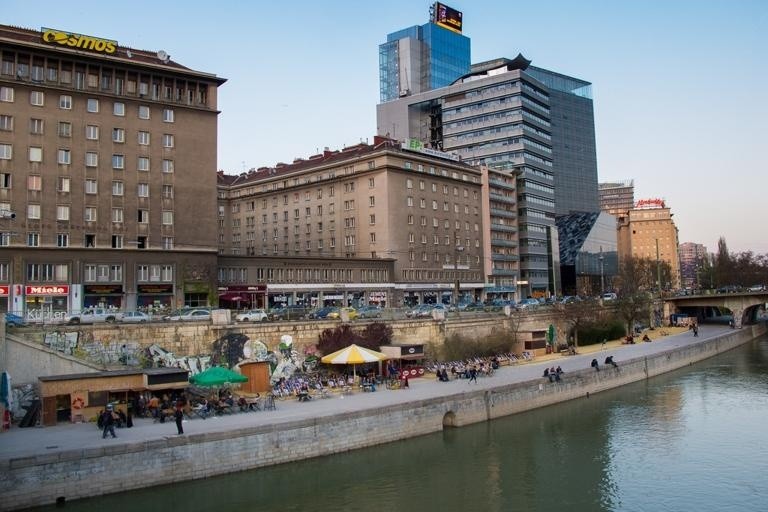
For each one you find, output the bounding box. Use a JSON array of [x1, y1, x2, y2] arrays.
[[661, 313, 687, 327]]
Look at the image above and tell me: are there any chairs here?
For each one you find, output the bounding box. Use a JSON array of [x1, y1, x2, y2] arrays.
[[422, 350, 532, 380], [265, 371, 377, 410], [97, 379, 261, 427]]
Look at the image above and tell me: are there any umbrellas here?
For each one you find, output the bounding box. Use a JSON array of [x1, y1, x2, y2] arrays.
[[188, 367, 248, 397], [321, 344, 388, 382]]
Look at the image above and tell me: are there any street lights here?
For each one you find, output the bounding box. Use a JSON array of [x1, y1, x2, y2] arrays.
[[693, 263, 702, 291], [598, 262, 608, 299]]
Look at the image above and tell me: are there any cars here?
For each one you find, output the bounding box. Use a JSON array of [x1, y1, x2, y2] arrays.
[[5, 312, 26, 327], [403, 294, 585, 319], [233, 305, 381, 323], [717, 282, 743, 294], [63, 307, 212, 324], [648, 285, 692, 298], [746, 283, 767, 293]]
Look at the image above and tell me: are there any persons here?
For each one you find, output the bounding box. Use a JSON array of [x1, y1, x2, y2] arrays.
[[435, 352, 499, 385], [274, 360, 398, 401], [543, 365, 565, 383], [693, 324, 698, 337], [643, 334, 651, 342], [591, 358, 600, 372], [95, 387, 261, 438], [604, 355, 618, 368]]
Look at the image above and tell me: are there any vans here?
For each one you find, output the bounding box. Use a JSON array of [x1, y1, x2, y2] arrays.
[[603, 293, 618, 302]]
